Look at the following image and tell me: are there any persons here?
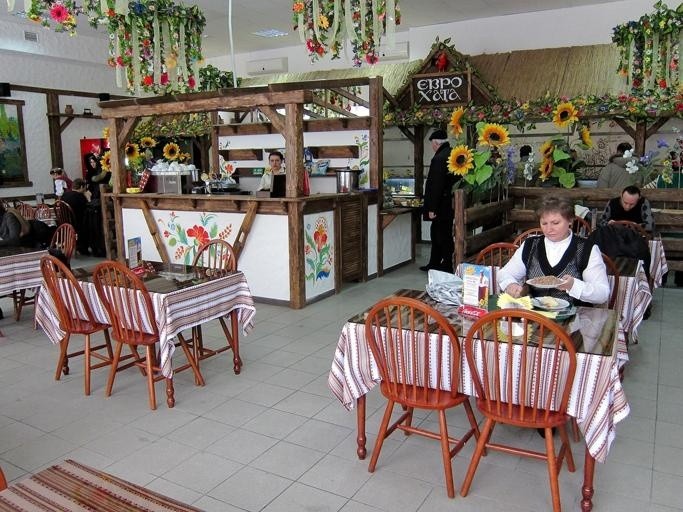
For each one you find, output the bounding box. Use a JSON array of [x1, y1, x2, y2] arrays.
[[257, 151, 290, 189], [478, 269, 487, 287], [419, 130, 454, 273], [597, 140, 652, 191], [511, 142, 537, 186], [496, 190, 611, 438], [49, 153, 109, 256], [0, 203, 32, 322], [601, 184, 655, 234]]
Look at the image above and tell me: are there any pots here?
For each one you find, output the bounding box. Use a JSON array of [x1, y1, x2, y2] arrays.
[[334, 167, 364, 192]]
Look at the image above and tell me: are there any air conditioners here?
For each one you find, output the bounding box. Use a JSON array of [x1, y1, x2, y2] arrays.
[[244, 56, 288, 76], [375, 41, 409, 62]]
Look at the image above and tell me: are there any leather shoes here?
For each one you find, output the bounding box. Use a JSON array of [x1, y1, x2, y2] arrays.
[[419, 265, 442, 271]]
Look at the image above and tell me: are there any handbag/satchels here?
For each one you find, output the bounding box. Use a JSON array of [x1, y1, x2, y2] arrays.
[[47, 248, 70, 271], [519, 276, 529, 296]]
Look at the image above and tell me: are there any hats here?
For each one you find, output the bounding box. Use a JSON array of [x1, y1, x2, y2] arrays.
[[520, 146, 531, 157], [429, 130, 447, 140]]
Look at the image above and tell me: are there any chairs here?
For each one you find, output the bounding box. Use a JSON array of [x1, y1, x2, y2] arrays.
[[0, 466, 8, 492]]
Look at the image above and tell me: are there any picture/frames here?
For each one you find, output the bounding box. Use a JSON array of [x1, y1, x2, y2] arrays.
[[0, 98, 33, 189]]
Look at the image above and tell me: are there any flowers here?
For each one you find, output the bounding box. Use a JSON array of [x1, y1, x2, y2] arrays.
[[99, 125, 191, 173], [6, 0, 208, 90], [448, 99, 513, 196], [524, 103, 592, 190], [291, 0, 402, 69], [610, 0, 683, 118], [622, 127, 683, 187]]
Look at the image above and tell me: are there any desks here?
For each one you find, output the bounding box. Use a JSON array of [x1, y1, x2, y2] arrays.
[[0, 458, 208, 512]]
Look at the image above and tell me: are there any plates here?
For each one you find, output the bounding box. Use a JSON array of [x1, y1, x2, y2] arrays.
[[531, 296, 570, 311], [526, 279, 566, 289]]
